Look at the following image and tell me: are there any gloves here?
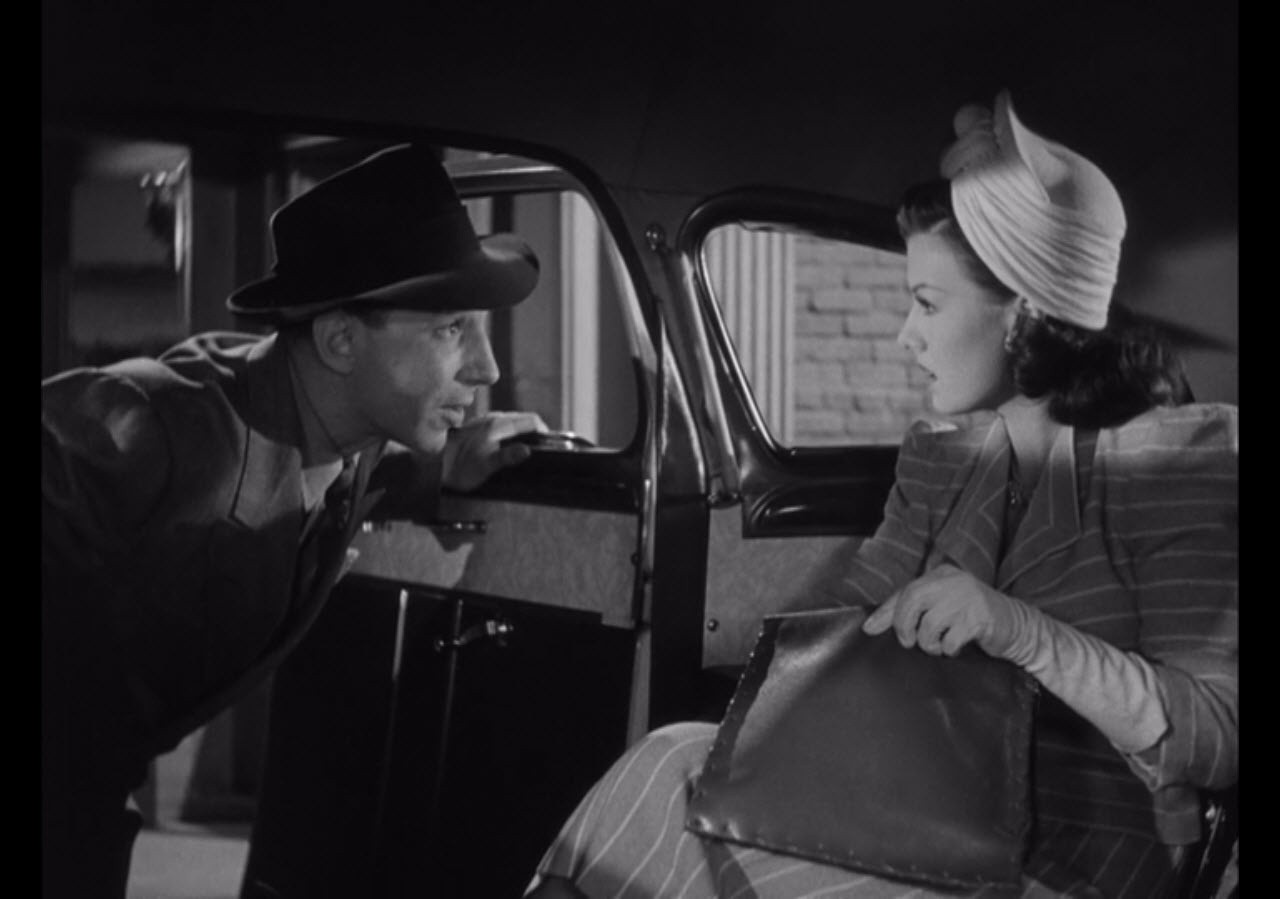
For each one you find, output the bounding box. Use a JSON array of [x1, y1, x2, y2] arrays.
[[862, 563, 1168, 753]]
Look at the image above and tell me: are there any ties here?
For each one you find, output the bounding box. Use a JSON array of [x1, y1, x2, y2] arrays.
[[289, 457, 361, 614]]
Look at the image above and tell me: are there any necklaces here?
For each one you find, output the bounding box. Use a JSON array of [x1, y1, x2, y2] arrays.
[[287, 349, 390, 486], [1007, 478, 1030, 511]]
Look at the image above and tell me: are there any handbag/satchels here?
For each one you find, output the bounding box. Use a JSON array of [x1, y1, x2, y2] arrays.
[[680, 609, 1036, 899]]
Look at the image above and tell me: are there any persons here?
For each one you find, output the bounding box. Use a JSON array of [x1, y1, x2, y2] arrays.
[[522, 89, 1239, 899], [39, 144, 553, 899]]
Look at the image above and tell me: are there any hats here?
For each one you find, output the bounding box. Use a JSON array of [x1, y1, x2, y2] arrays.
[[938, 86, 1128, 337], [226, 141, 540, 324]]
